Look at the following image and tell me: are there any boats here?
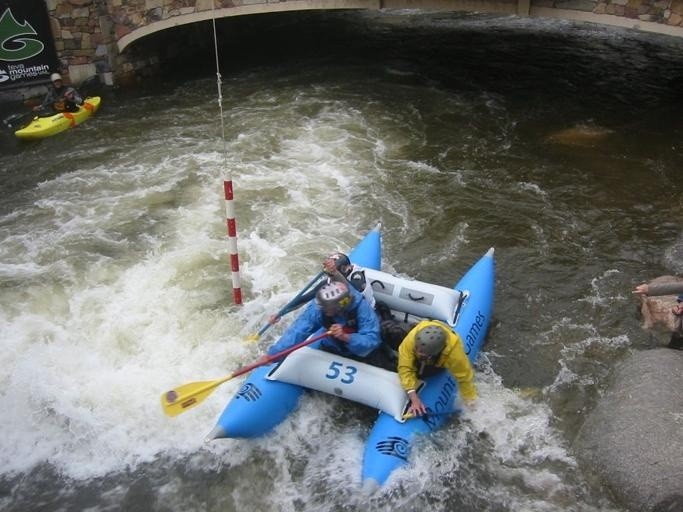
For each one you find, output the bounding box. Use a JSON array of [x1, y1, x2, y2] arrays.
[[205, 223, 495, 500], [13, 95, 101, 141]]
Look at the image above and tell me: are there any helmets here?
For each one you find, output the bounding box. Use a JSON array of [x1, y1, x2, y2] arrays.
[[313, 281, 348, 307], [328, 253, 350, 274], [50, 72, 62, 83], [411, 325, 448, 362]]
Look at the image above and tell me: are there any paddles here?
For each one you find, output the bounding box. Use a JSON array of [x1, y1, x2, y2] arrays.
[[3, 92, 73, 126], [160, 330, 334, 418], [246, 271, 326, 344]]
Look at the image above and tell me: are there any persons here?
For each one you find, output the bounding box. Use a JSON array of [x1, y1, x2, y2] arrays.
[[32, 72, 83, 116], [376, 297, 476, 418], [631, 281, 683, 351], [257, 282, 390, 369], [268, 253, 376, 325]]
